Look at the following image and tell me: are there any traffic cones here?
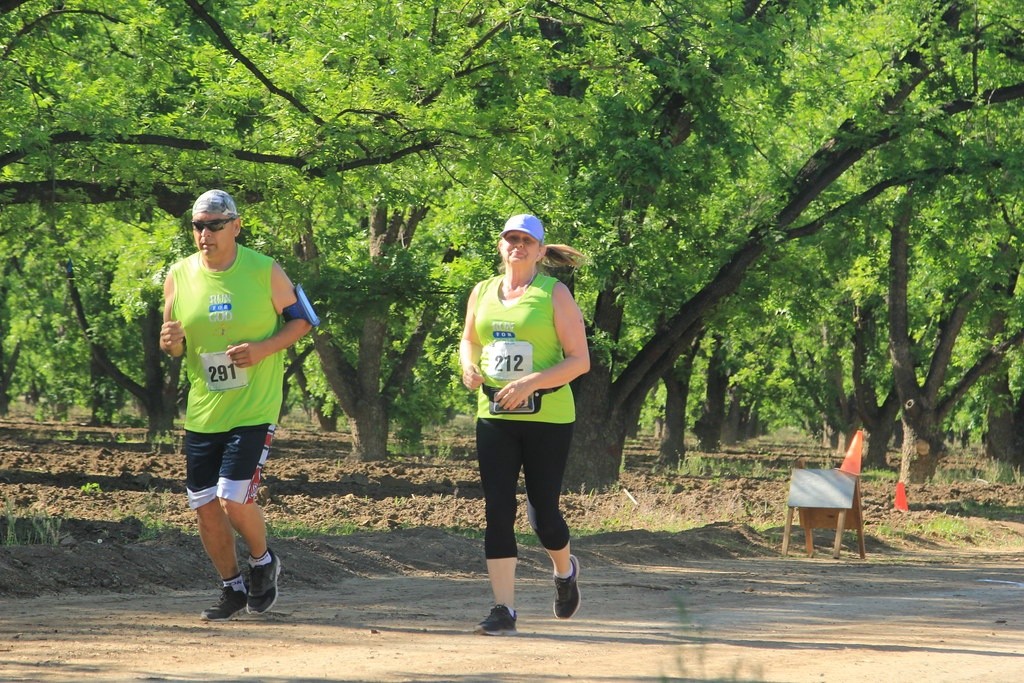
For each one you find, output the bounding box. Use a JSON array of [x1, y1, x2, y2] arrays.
[[837, 430, 865, 476], [894, 482, 908, 509]]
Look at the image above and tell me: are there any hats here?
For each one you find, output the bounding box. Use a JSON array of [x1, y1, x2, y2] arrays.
[[192, 189, 238, 219], [499, 214, 544, 247]]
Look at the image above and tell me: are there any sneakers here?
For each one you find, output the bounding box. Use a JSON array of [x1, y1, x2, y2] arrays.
[[476, 604, 518, 635], [246, 547, 281, 615], [200, 582, 249, 621], [553, 554, 581, 619]]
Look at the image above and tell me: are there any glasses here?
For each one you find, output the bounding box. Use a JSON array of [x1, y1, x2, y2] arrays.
[[191, 215, 237, 232]]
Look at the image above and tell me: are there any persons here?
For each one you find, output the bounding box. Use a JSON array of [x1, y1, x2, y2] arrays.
[[160, 189, 321, 622], [460, 214, 591, 637]]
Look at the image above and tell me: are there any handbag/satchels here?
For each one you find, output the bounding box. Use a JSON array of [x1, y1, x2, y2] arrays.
[[480, 383, 566, 415]]
